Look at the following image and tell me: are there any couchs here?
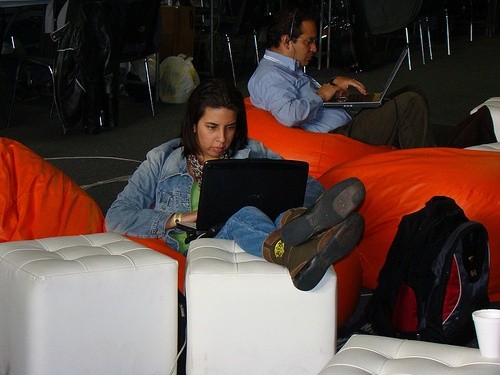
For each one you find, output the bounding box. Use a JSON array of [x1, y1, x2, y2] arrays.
[[243, 95, 395, 168]]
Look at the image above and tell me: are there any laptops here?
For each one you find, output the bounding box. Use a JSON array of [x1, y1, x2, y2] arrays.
[[323, 48, 408, 106], [176, 158, 309, 235]]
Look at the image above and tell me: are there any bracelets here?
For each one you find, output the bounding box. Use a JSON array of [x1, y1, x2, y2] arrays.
[[329, 76, 336, 86], [174, 213, 182, 226]]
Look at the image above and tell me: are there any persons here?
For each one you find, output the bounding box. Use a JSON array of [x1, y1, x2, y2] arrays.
[[248, 15, 435, 149], [105, 79, 365, 291]]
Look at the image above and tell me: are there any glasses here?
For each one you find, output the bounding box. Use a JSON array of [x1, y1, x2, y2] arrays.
[[296, 36, 318, 46]]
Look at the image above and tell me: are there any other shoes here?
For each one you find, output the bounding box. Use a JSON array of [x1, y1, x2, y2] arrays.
[[106, 62, 138, 103]]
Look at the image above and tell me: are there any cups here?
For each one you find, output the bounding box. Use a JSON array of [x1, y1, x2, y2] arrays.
[[472, 308, 500, 359]]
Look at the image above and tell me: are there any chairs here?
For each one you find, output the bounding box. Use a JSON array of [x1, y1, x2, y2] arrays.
[[192, 0, 500, 137], [7, 0, 163, 137]]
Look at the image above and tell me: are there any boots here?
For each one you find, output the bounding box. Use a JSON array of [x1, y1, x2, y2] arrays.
[[280, 177, 366, 247], [263, 214, 364, 291]]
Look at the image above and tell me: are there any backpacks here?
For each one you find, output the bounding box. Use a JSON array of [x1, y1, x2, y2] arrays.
[[338, 196, 490, 345]]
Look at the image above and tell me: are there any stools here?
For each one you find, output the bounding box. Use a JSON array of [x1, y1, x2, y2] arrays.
[[0, 233, 179, 375], [184, 238, 338, 375], [317, 334, 500, 375]]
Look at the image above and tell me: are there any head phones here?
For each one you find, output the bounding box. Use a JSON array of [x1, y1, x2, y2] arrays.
[[285, 8, 299, 42]]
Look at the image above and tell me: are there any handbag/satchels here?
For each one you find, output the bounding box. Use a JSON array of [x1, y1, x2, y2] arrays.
[[159, 54, 201, 104], [130, 54, 157, 86]]
